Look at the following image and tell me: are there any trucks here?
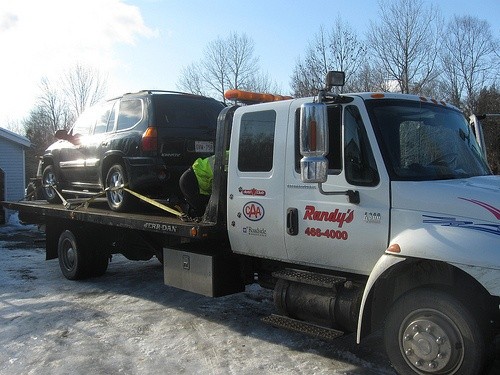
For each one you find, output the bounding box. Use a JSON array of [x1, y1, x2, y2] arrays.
[[0, 70, 500, 374]]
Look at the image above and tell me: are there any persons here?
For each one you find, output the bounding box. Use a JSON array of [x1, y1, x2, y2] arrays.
[[179, 151, 230, 215]]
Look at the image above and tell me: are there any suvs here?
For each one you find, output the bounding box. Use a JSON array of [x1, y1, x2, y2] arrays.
[[39, 88, 226, 211]]
